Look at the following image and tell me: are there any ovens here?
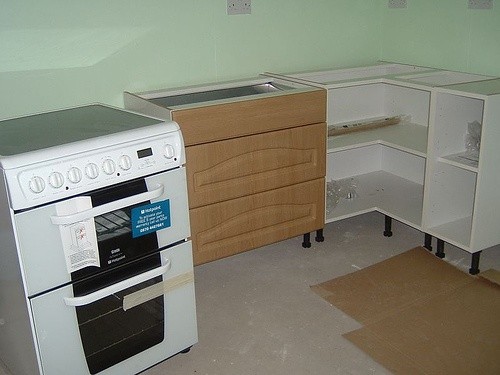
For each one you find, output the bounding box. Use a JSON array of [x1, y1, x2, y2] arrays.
[[0, 101, 199, 375]]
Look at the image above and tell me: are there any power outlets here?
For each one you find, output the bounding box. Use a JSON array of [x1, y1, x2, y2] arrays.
[[388, 0, 408, 9], [468, 0, 493, 10]]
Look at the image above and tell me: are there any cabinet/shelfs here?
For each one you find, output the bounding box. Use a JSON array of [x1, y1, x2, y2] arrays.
[[127, 73, 325, 267], [265, 61, 500, 274]]
